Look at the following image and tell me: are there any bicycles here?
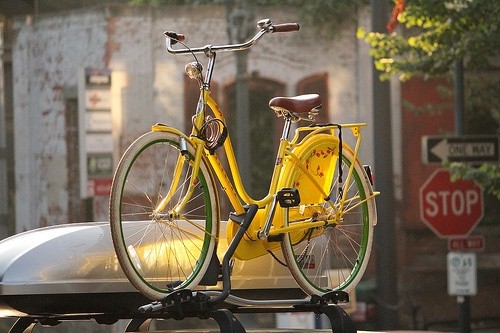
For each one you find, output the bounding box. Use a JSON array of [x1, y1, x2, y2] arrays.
[[108, 18, 382, 302]]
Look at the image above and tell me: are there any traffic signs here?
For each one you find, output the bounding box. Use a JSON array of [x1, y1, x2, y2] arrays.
[[420, 135, 498, 163]]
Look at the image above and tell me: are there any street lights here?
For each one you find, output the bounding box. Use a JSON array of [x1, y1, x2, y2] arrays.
[[228, 0, 259, 210]]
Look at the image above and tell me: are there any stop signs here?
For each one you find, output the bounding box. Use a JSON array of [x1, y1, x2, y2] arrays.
[[418, 167, 486, 238]]
